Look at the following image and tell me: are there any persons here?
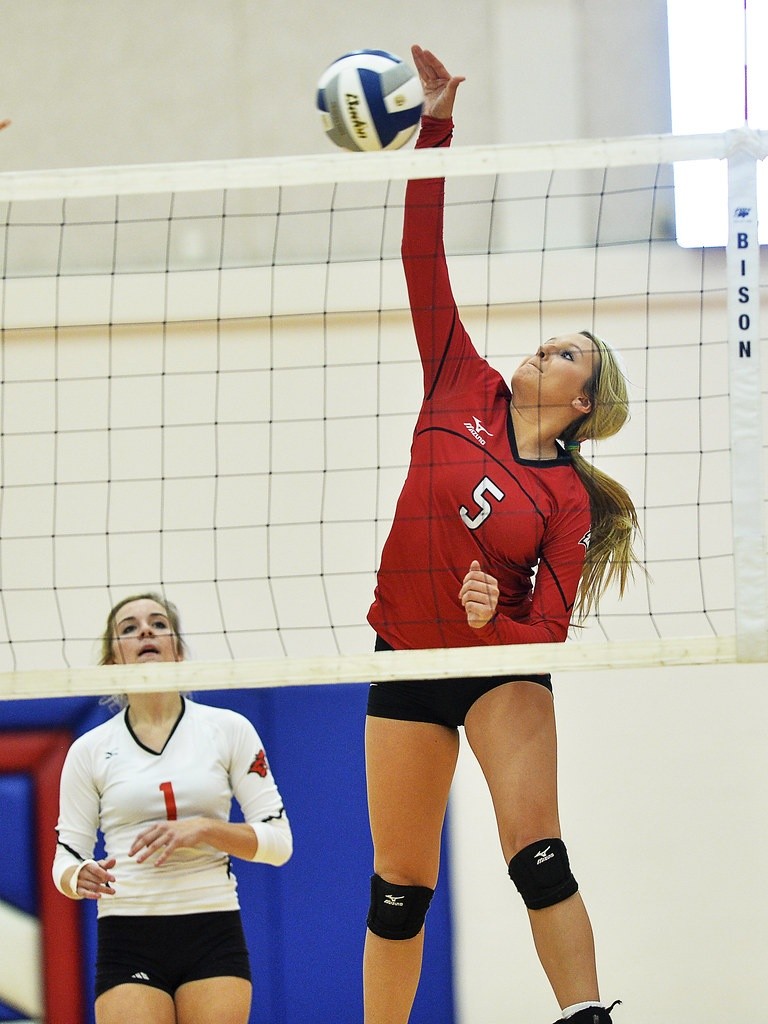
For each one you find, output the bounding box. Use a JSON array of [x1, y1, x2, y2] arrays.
[[51, 593, 293, 1024], [362, 46, 640, 1024]]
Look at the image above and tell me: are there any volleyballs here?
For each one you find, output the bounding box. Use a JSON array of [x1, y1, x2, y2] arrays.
[[315, 49, 424, 153]]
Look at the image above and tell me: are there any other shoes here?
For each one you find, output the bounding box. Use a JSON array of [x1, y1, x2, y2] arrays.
[[553, 1000, 621, 1024]]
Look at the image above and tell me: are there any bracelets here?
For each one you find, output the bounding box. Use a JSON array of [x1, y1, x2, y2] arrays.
[[70, 859, 99, 897]]
